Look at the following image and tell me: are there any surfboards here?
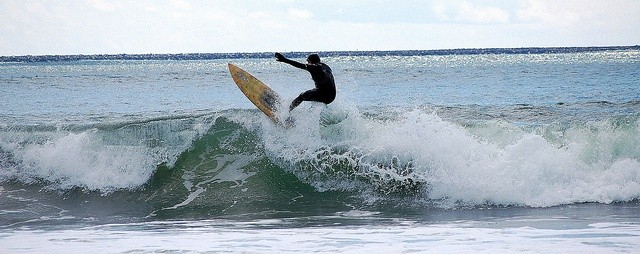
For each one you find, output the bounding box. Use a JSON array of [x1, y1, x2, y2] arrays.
[[228, 63, 296, 130]]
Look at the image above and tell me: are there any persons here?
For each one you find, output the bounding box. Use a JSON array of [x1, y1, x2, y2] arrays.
[[275, 52, 336, 111]]
